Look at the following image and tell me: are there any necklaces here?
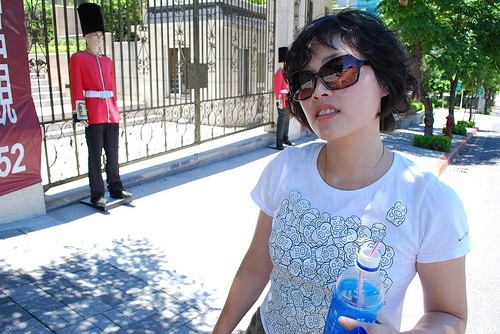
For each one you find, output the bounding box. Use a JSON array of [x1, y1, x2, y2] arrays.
[[325, 140, 385, 190]]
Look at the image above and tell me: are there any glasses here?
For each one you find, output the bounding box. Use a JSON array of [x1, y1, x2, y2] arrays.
[[287, 53, 371, 101]]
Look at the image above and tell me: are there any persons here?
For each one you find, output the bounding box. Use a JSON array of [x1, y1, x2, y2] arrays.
[[273, 47, 292, 151], [69, 3, 135, 207], [212, 12, 472, 334]]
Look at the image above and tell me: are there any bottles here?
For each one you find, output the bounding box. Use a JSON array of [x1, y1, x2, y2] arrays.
[[322, 247, 385, 334]]
